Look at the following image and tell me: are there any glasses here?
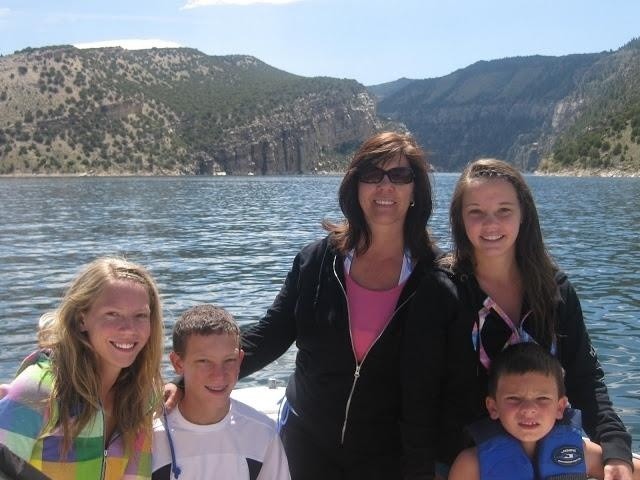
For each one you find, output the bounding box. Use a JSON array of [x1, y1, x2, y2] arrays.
[[357, 167, 414, 184]]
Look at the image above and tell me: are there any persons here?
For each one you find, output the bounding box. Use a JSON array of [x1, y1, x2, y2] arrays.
[[0, 252, 167, 479], [146, 301, 293, 479], [442, 340, 640, 480], [389, 157, 634, 480], [155, 129, 466, 480]]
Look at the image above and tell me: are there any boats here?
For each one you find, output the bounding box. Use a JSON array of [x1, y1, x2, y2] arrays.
[[230, 378, 289, 422]]
